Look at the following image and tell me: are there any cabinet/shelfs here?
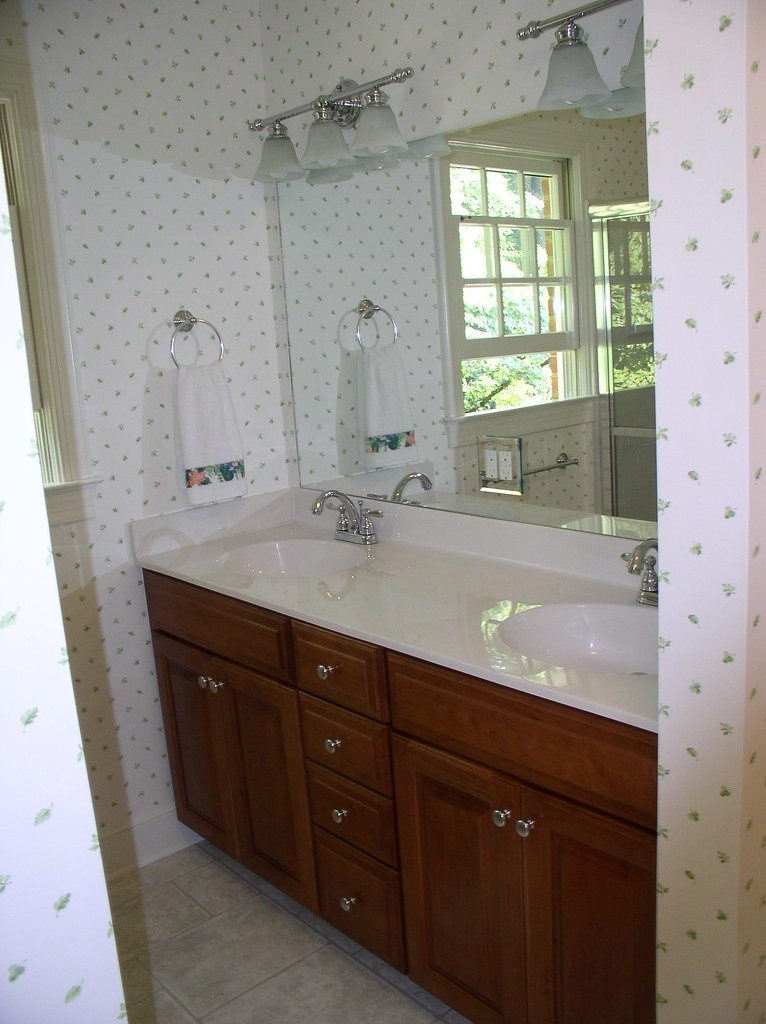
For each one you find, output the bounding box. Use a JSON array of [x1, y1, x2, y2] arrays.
[[385, 648, 658, 1024], [288, 616, 409, 978], [141, 569, 319, 916]]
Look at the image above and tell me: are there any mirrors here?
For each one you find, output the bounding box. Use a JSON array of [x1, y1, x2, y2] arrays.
[[276, 87, 657, 541]]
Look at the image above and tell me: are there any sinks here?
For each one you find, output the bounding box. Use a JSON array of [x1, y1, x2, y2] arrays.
[[496, 601, 659, 680], [215, 538, 369, 580]]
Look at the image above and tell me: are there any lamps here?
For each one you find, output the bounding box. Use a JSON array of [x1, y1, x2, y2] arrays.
[[305, 135, 452, 183], [247, 66, 415, 183], [581, 89, 645, 119], [517, 0, 645, 109]]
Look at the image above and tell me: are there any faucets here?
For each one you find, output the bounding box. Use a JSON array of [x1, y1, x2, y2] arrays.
[[619, 537, 658, 608], [389, 470, 434, 504], [310, 488, 385, 547]]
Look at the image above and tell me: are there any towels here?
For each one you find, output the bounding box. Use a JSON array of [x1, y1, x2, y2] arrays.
[[352, 343, 421, 470], [171, 360, 250, 509]]
